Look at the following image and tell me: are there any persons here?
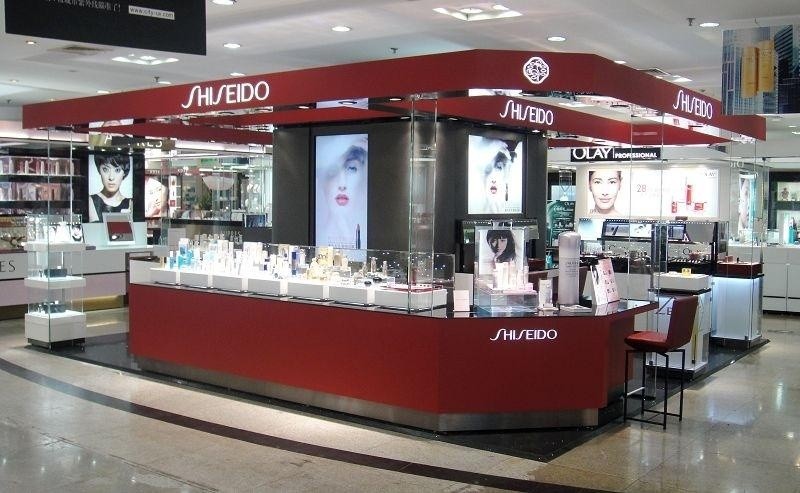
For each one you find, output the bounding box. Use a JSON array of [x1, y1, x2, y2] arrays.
[[473, 135, 520, 212], [588, 169, 623, 217], [591, 264, 600, 283], [484, 228, 517, 264], [145, 173, 169, 217], [88, 153, 133, 222], [739, 175, 751, 229], [317, 134, 369, 249], [72, 224, 83, 243]]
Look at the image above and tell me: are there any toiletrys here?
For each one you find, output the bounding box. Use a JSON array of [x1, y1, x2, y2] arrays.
[[160, 229, 419, 288], [0, 155, 79, 248], [490, 260, 535, 292], [557, 230, 580, 306]]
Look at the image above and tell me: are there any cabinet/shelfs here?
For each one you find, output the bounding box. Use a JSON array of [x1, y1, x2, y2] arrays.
[[601, 219, 730, 275], [0, 146, 454, 319], [728, 247, 800, 313]]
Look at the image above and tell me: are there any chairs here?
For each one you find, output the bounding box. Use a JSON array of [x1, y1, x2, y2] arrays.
[[623, 295, 698, 429]]
